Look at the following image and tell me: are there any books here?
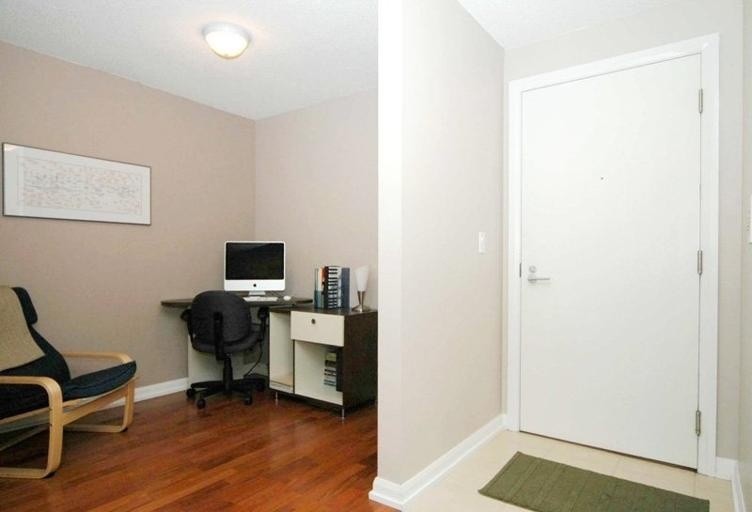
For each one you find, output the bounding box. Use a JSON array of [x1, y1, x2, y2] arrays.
[[324, 350, 337, 386]]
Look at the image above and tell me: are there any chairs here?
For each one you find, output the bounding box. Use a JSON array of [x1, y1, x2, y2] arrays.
[[180, 291, 270, 409], [0, 282, 141, 481]]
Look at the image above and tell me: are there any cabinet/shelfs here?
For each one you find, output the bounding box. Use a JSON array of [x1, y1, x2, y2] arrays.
[[269, 303, 377, 420]]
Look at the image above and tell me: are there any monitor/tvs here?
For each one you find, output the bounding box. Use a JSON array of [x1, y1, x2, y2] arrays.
[[223, 241, 286, 296]]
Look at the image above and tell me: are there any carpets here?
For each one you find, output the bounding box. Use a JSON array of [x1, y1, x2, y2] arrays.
[[478, 451, 710, 512]]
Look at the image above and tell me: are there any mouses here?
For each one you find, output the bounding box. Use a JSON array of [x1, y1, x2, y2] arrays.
[[283, 295, 291, 301]]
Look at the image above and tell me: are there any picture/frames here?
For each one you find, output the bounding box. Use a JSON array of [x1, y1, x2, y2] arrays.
[[2, 143, 151, 225]]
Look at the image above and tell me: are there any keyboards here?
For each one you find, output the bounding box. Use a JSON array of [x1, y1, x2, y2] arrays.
[[243, 296, 278, 303]]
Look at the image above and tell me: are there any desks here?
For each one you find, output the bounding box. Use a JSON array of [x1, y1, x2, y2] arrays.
[[161, 295, 313, 390]]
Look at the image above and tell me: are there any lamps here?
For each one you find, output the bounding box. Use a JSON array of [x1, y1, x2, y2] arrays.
[[203, 21, 250, 58], [351, 265, 371, 313]]
